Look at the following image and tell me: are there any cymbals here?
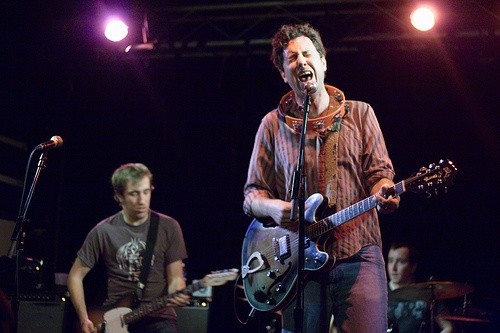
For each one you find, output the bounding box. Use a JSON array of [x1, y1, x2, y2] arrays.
[[388, 279, 478, 303], [435, 314, 486, 324]]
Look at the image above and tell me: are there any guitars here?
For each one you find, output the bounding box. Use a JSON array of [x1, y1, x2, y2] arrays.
[[240, 158, 459, 313], [72, 263, 239, 333]]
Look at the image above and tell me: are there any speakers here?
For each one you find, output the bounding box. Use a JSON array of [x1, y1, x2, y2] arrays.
[[174, 305, 209, 333], [16, 301, 65, 333]]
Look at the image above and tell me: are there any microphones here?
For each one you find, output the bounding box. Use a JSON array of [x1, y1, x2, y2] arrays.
[[34, 135, 64, 150], [304, 79, 319, 93]]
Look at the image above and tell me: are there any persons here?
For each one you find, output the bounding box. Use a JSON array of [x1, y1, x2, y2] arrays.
[[386, 240, 455, 333], [242, 24, 400, 333], [67, 162, 190, 333]]
